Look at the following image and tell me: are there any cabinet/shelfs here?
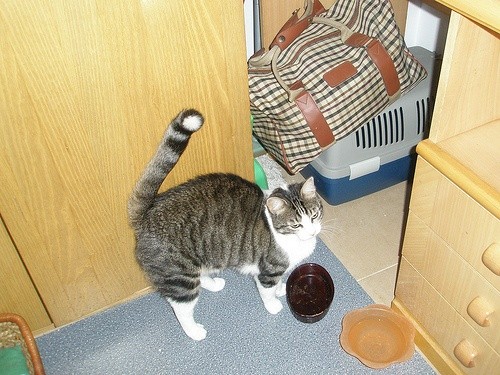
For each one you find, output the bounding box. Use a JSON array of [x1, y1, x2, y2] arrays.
[[0, 1, 257, 334]]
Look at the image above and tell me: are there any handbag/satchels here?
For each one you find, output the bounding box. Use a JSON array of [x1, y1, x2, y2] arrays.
[[247, 0, 428, 176]]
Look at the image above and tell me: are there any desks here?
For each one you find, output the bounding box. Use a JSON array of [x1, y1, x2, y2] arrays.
[[258, 0, 500, 375]]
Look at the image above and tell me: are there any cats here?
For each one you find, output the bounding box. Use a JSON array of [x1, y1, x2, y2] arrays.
[[126, 107, 337, 342]]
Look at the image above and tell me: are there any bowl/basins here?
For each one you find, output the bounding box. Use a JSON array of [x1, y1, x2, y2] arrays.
[[286, 263, 335, 324], [340, 303, 416, 370]]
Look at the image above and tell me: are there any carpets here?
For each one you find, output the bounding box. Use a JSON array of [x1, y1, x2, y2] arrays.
[[30, 227, 436, 375]]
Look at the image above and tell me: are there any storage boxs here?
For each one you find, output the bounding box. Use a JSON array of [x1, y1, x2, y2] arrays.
[[299, 46, 444, 206]]
